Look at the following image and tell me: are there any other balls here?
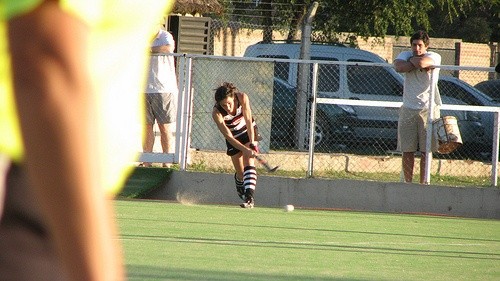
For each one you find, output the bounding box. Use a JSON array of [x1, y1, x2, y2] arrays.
[[286, 204, 295, 212]]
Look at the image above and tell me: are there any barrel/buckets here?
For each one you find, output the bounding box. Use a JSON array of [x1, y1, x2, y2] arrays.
[[432, 116, 463, 154]]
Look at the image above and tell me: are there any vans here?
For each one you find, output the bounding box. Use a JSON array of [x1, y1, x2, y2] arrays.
[[242, 39, 484, 158]]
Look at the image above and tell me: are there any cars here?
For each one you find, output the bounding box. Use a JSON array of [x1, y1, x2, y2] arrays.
[[437, 73, 500, 145], [475, 79, 500, 106], [271, 78, 359, 152]]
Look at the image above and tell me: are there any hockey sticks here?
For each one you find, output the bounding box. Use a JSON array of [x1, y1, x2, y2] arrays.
[[255, 154, 280, 173]]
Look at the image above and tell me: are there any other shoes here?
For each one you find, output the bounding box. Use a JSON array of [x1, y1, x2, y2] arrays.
[[163, 163, 171, 168], [236, 186, 245, 199], [240, 197, 254, 208], [139, 162, 153, 168]]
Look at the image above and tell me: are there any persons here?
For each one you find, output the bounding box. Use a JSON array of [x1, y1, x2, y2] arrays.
[[393, 31, 442, 183], [0, 0, 175, 281], [139, 30, 175, 166], [212, 82, 262, 208]]
